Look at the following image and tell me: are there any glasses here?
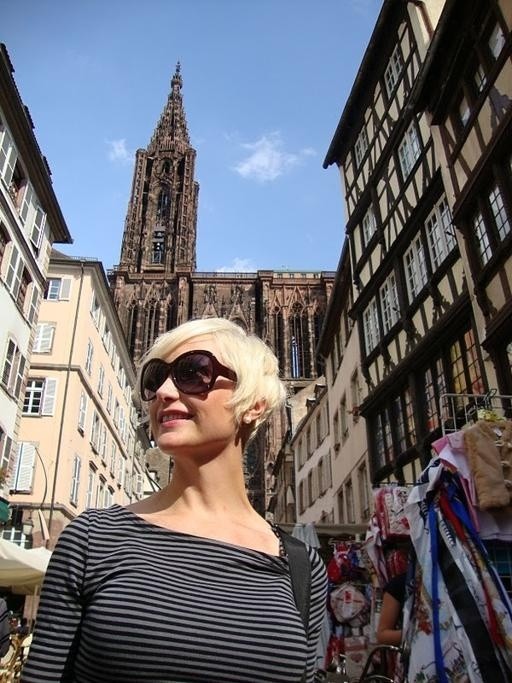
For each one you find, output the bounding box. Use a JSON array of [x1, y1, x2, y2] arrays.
[[140, 350, 236, 402]]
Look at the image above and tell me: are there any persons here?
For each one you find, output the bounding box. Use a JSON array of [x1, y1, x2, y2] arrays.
[[377, 575, 407, 645], [18, 318, 328, 683], [18, 616, 28, 637]]
